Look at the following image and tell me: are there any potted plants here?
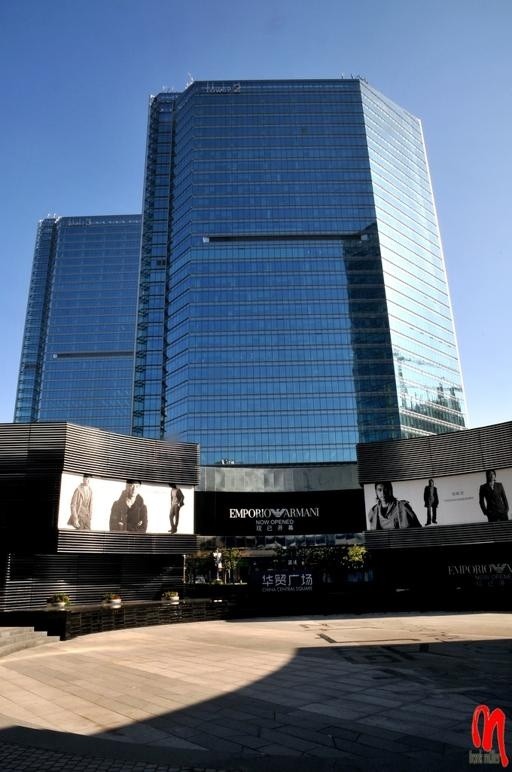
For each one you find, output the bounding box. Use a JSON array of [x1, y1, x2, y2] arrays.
[[160, 590, 182, 606], [100, 592, 125, 608], [46, 593, 72, 613]]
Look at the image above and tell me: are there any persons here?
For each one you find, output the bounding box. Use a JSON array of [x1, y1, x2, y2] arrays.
[[109, 479, 148, 533], [478, 469, 509, 522], [168, 483, 185, 534], [67, 473, 93, 530], [423, 479, 439, 525], [367, 482, 422, 531]]
